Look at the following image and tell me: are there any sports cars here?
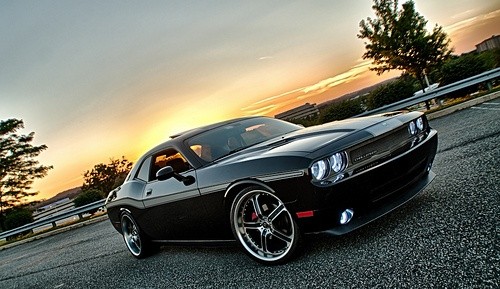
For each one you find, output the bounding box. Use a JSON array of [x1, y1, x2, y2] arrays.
[[106, 110, 438, 266]]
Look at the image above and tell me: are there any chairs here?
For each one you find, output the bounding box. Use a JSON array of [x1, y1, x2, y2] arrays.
[[166, 158, 189, 175], [239, 128, 267, 147]]
